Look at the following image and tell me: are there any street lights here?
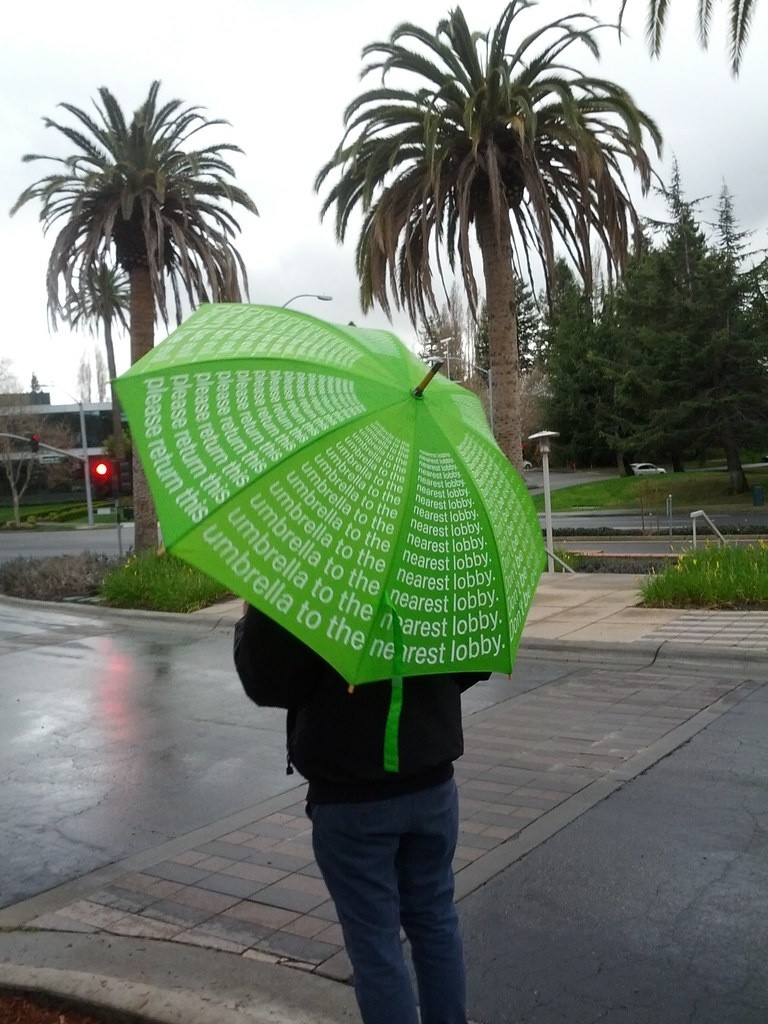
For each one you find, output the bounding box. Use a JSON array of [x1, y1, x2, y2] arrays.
[[427, 337, 493, 436], [29, 383, 94, 530], [529, 430, 559, 573]]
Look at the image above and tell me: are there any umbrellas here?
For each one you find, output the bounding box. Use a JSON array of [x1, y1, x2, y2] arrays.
[[110, 302, 546, 772]]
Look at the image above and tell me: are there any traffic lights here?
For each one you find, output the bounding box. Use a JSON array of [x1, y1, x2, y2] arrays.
[[91, 460, 110, 479], [30, 436, 40, 451]]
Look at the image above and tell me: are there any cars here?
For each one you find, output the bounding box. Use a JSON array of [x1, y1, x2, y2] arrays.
[[522, 461, 533, 468], [631, 463, 667, 477]]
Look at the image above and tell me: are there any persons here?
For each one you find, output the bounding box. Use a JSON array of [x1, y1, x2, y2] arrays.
[[234, 580, 493, 1023]]
